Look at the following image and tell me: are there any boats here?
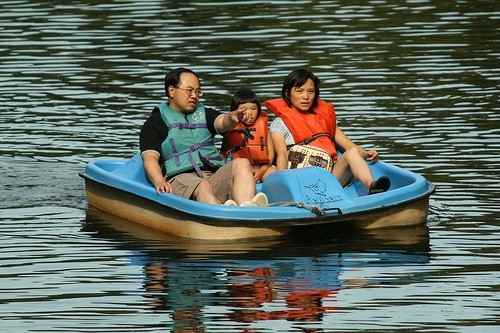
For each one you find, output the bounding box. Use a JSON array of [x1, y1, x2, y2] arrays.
[[81, 139, 435, 254]]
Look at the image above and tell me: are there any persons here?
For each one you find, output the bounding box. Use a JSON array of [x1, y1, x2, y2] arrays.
[[140, 68, 268, 208], [264, 69, 391, 195], [220, 89, 276, 183]]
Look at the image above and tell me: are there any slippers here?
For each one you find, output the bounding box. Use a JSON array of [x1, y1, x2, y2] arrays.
[[368, 176, 391, 196]]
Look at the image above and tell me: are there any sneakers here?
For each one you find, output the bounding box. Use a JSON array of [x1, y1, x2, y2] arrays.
[[224, 192, 269, 207]]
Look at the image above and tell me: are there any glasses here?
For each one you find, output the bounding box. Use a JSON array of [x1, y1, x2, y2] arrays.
[[175, 86, 204, 97]]
